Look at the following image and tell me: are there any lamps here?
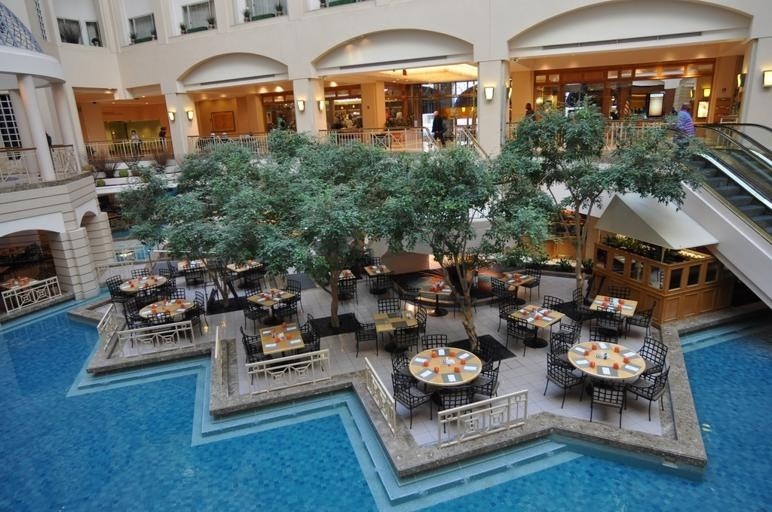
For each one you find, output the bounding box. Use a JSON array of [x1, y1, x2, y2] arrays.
[[702, 88, 710, 98], [483, 86, 495, 101], [689, 89, 695, 98], [736, 73, 746, 88], [316, 100, 326, 112], [506, 86, 513, 100], [187, 110, 195, 121], [297, 97, 306, 112], [168, 111, 176, 121], [761, 68, 772, 88]]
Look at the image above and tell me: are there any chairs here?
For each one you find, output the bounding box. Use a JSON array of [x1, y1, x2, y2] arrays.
[[102, 242, 674, 435]]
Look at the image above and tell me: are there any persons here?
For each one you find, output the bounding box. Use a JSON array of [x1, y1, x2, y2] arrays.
[[525, 102, 536, 122], [158, 127, 166, 152], [672, 102, 695, 149], [431, 110, 446, 148], [130, 130, 142, 156]]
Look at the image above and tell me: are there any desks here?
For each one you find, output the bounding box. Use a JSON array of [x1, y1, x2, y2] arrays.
[[1, 243, 48, 308]]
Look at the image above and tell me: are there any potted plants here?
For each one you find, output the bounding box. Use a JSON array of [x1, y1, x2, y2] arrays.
[[89, 1, 327, 47]]
